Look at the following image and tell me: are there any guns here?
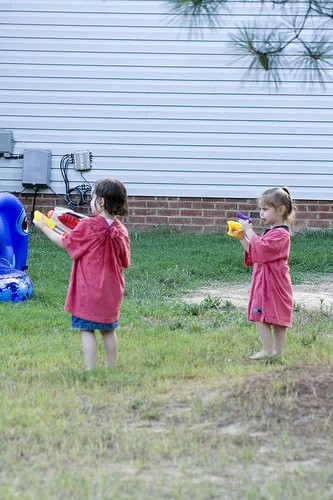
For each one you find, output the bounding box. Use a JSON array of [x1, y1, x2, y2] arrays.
[[34, 204, 90, 236], [226, 220, 245, 237], [236, 212, 252, 225]]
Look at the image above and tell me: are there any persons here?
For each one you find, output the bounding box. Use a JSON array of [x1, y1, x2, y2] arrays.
[[234, 187, 293, 359], [33, 178, 130, 371]]
